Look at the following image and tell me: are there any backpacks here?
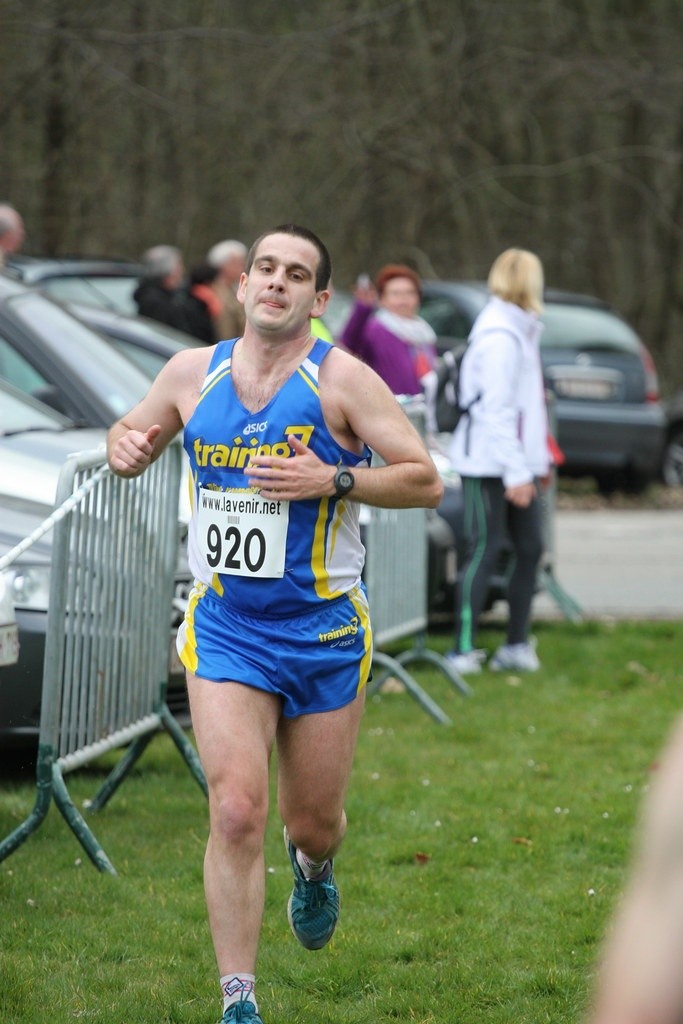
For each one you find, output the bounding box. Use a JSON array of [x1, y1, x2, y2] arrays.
[[420, 341, 480, 452]]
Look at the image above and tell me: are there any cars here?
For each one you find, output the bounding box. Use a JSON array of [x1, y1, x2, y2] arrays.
[[0, 494, 162, 738], [0, 379, 195, 678], [421, 279, 669, 500], [7, 254, 142, 319], [55, 297, 213, 380], [0, 263, 157, 428]]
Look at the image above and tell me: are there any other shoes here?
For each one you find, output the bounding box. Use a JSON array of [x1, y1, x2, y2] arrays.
[[491, 633, 541, 672], [447, 649, 487, 674]]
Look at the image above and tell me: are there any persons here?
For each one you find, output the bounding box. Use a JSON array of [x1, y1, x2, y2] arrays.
[[107, 224, 443, 1024], [133, 239, 335, 342], [344, 263, 443, 603], [0, 204, 26, 272], [589, 723, 683, 1024], [450, 248, 551, 670]]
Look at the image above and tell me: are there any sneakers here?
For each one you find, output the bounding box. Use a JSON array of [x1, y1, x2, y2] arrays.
[[220, 981, 264, 1024], [284, 825, 339, 950]]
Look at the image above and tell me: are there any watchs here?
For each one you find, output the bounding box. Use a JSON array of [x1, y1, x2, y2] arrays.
[[329, 466, 356, 501]]
